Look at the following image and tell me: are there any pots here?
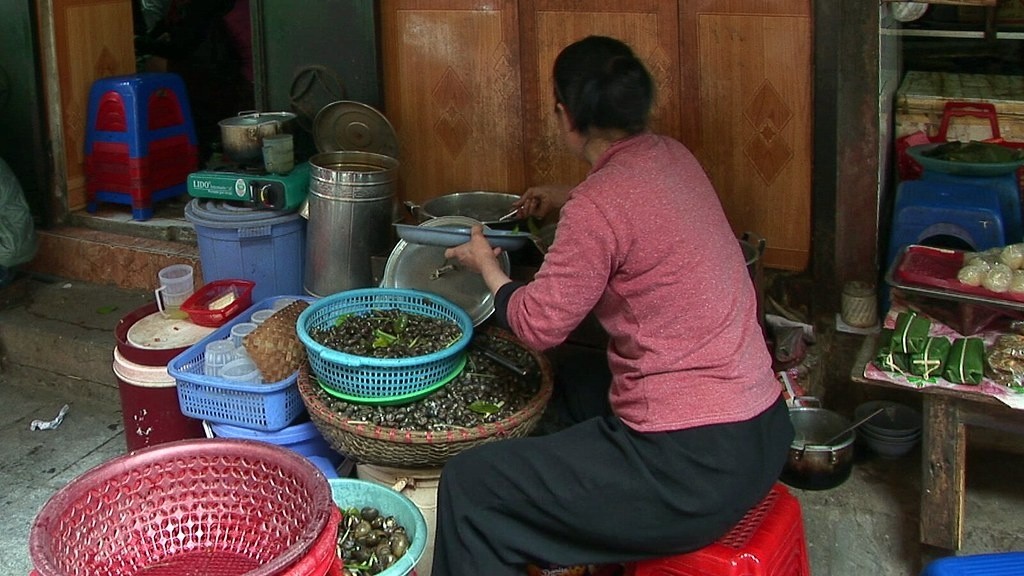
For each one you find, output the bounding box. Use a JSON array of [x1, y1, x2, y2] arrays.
[[218, 109, 300, 173], [779, 396, 859, 490], [735, 230, 765, 279]]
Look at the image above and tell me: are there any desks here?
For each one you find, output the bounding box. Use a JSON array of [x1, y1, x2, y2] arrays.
[[851, 293, 1023, 552]]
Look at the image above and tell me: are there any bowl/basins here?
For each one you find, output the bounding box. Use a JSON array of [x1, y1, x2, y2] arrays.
[[858, 399, 924, 456], [326, 476, 428, 576]]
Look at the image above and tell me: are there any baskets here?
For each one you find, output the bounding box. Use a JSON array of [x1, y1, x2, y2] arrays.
[[29, 277, 555, 576]]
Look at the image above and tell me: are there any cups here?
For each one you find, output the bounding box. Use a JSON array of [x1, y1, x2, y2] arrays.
[[205, 298, 297, 384], [154, 264, 195, 321], [262, 134, 294, 175]]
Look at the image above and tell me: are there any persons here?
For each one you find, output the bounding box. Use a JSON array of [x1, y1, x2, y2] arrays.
[[430, 35, 790, 576], [0, 156, 40, 288]]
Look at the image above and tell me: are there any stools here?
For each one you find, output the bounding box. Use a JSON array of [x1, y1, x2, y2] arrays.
[[623, 481, 811, 575], [80, 66, 200, 222]]
